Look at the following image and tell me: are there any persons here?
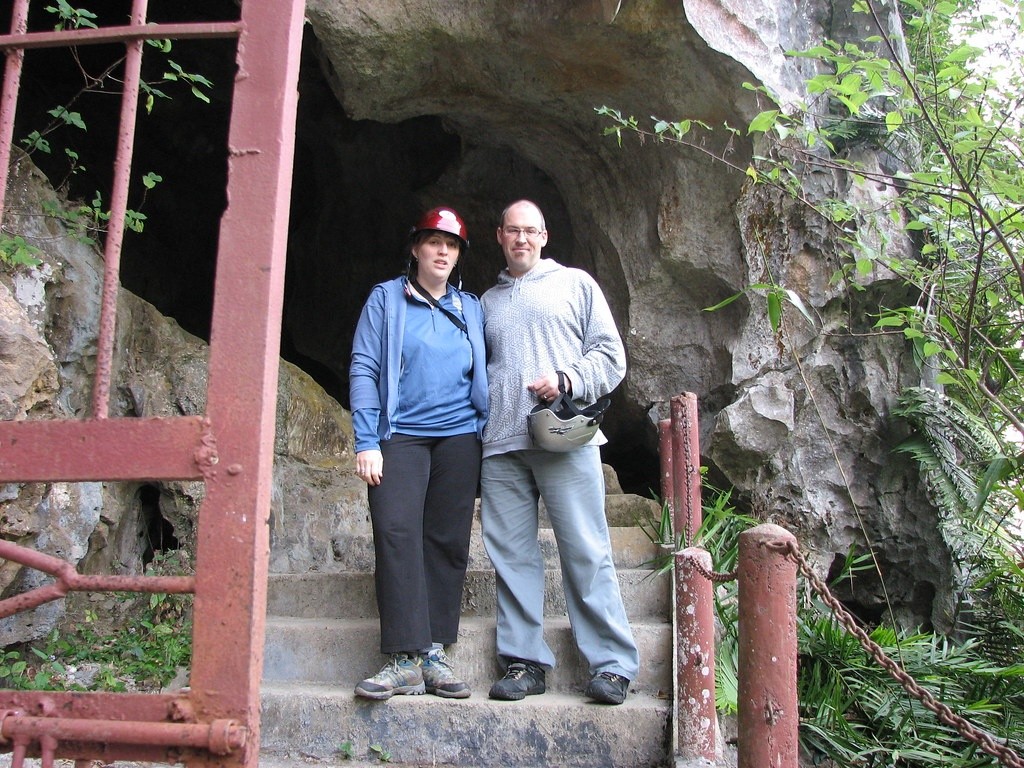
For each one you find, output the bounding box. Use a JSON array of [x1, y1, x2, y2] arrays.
[[480, 201, 639, 705], [349, 207, 491, 700]]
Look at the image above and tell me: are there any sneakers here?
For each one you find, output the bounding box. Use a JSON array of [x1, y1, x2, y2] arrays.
[[585, 671, 630, 704], [352, 651, 426, 701], [486, 659, 546, 701], [420, 644, 473, 699]]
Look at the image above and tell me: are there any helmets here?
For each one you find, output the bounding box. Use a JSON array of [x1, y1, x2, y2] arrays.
[[527, 398, 611, 454], [411, 208, 470, 247]]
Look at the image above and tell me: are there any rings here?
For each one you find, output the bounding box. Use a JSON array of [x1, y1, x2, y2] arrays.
[[543, 394, 547, 398]]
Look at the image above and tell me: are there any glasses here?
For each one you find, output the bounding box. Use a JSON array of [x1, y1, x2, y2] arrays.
[[502, 227, 543, 237]]
